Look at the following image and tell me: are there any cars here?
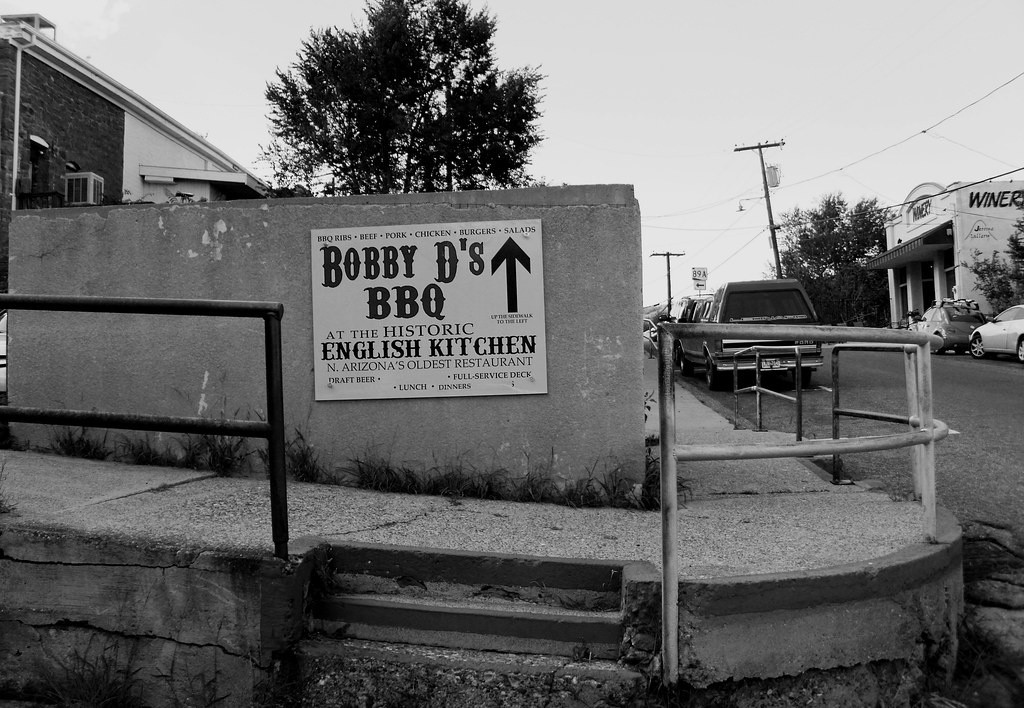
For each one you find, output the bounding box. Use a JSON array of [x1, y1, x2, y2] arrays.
[[969, 304, 1024, 363]]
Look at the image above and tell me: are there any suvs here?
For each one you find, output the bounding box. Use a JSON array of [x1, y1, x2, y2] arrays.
[[906, 299, 988, 356], [657, 293, 716, 365]]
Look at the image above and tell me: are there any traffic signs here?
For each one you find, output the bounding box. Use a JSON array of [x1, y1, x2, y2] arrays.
[[693, 268, 708, 290], [312, 217, 548, 401]]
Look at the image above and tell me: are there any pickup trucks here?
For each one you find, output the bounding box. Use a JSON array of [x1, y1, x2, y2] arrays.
[[680, 277, 824, 391]]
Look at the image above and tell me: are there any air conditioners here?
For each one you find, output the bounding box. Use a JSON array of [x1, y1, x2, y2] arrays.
[[63, 172, 104, 207]]
